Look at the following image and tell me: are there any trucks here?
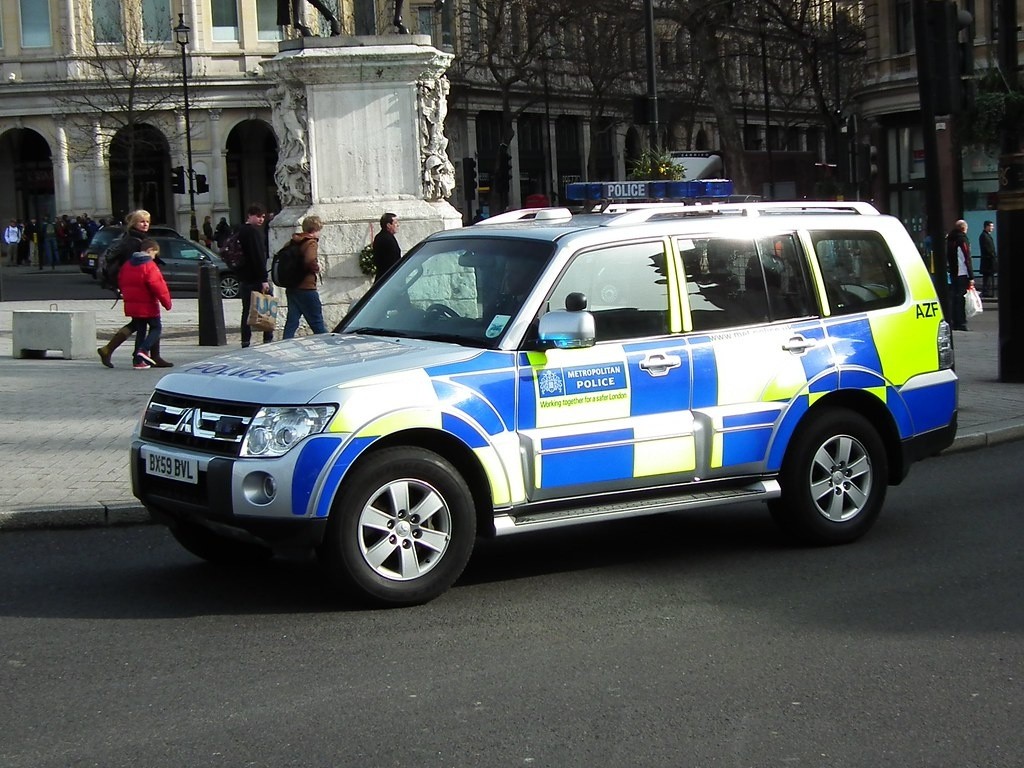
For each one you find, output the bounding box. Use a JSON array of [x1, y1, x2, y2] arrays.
[[664, 150, 818, 201]]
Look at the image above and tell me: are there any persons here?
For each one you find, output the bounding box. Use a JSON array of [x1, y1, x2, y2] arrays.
[[949, 219, 975, 331], [119, 239, 172, 369], [264, 214, 273, 259], [979, 220, 998, 298], [213, 217, 231, 252], [473, 209, 483, 224], [5, 213, 124, 270], [203, 216, 213, 249], [373, 213, 410, 315], [505, 259, 535, 304], [97, 208, 174, 368], [283, 216, 329, 340], [236, 204, 274, 348]]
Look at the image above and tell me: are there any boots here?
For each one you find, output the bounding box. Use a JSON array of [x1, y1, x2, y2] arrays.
[[97, 326, 132, 369], [150, 337, 174, 368]]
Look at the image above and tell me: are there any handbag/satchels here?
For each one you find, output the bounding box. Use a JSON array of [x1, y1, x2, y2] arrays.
[[247, 291, 279, 331], [963, 285, 983, 321]]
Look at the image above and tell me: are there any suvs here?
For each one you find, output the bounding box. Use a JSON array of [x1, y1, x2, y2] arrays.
[[128, 172, 961, 611]]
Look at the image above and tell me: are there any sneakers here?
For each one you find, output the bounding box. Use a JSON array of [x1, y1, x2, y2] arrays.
[[133, 348, 156, 369]]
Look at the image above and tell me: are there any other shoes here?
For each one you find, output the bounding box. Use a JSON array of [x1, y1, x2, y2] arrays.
[[951, 325, 974, 331]]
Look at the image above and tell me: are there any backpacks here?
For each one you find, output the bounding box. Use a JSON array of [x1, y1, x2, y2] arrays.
[[221, 225, 258, 269], [78, 227, 88, 240], [102, 233, 142, 309], [1, 226, 19, 245], [56, 227, 65, 239], [46, 223, 55, 237], [267, 238, 323, 288]]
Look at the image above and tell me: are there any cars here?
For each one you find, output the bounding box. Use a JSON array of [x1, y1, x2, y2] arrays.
[[96, 235, 242, 299], [80, 223, 180, 273]]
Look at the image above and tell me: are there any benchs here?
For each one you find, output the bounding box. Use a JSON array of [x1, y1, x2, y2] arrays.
[[12, 309, 97, 360]]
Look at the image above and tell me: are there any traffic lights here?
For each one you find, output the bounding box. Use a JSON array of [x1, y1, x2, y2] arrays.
[[170, 166, 185, 194], [196, 174, 209, 194]]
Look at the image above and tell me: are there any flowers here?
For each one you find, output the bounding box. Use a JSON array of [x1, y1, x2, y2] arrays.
[[358, 244, 378, 278], [629, 146, 687, 180]]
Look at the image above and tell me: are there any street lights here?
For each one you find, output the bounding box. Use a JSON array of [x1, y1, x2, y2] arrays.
[[755, 14, 776, 196], [538, 52, 555, 207], [174, 11, 201, 244]]
